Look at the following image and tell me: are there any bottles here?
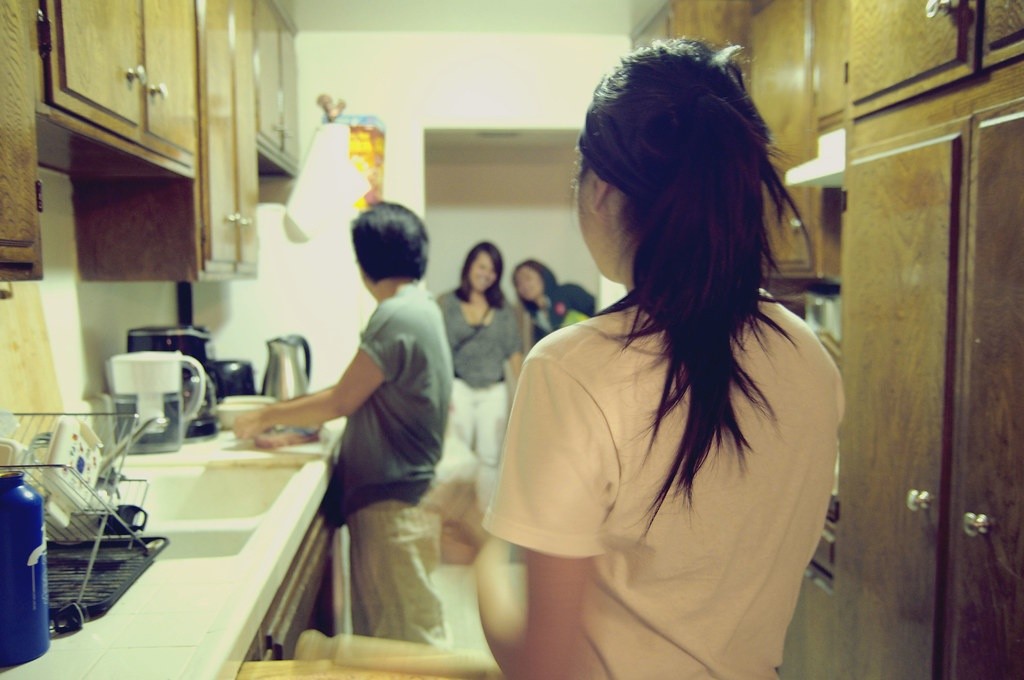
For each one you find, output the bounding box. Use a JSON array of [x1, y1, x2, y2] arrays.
[[0, 471, 49, 671]]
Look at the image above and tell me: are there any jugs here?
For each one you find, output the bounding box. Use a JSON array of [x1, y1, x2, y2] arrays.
[[104, 353, 206, 453], [261, 334, 310, 403]]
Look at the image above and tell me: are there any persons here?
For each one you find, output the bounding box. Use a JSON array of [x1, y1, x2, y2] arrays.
[[232, 202, 452, 646], [511, 258, 598, 345], [433, 240, 525, 470], [426, 39, 847, 680]]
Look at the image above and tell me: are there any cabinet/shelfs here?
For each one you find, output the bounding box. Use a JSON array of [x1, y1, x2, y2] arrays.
[[256, 0, 300, 179], [837, 83, 1024, 680], [845, 0, 1024, 140], [73, 0, 259, 282], [34, 0, 197, 183], [0, 0, 44, 282]]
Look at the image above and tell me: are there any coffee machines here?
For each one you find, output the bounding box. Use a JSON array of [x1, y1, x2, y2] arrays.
[[125, 324, 217, 443]]
